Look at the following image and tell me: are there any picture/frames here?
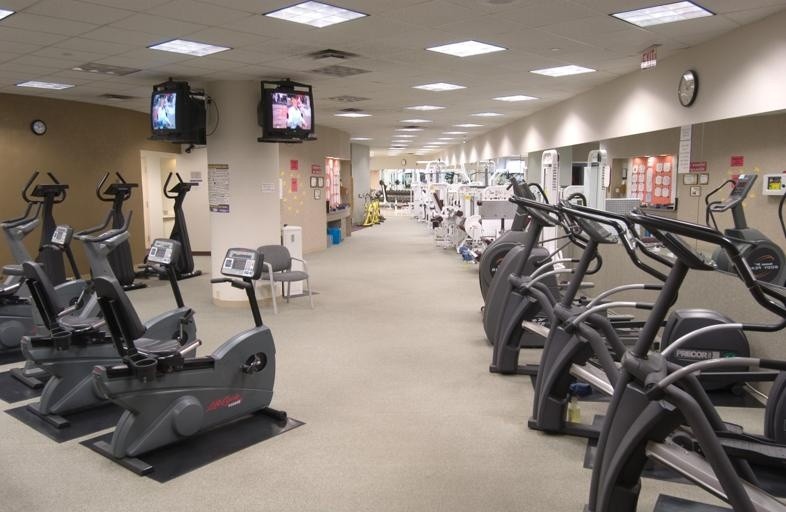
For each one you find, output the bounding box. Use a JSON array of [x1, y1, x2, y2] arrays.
[[690, 186, 701, 197], [310, 175, 318, 188], [317, 176, 324, 188], [314, 189, 321, 200], [699, 173, 709, 186], [683, 172, 698, 185]]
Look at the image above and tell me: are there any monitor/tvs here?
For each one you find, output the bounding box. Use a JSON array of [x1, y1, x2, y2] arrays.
[[257, 88, 313, 138], [150, 90, 183, 136]]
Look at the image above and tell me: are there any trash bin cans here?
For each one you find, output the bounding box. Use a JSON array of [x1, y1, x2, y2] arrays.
[[327, 227, 341, 244]]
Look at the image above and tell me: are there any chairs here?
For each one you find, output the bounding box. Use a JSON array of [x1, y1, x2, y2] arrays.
[[255, 244, 314, 313]]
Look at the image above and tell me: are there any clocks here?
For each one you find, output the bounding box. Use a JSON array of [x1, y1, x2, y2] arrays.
[[677, 69, 698, 107], [30, 119, 48, 136]]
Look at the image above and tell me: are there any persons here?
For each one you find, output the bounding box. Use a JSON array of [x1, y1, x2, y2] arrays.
[[288, 96, 307, 129], [157, 99, 172, 126]]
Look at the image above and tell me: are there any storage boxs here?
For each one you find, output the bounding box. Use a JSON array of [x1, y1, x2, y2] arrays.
[[328, 226, 340, 243], [327, 234, 334, 248]]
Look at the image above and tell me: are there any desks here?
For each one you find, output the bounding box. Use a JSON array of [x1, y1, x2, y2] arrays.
[[327, 205, 351, 240]]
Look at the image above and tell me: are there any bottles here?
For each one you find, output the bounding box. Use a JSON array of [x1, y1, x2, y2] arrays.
[[568, 397, 581, 422]]
